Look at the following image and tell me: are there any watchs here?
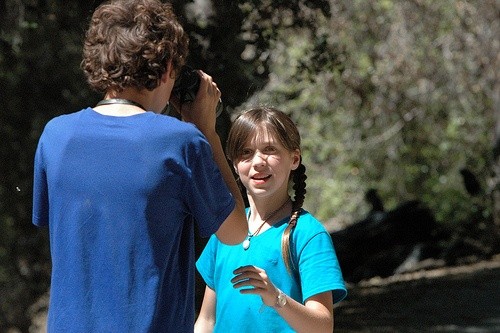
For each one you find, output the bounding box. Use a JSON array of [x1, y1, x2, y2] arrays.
[[272, 288, 287, 310]]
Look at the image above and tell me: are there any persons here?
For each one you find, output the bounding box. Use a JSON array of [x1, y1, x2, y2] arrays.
[[192, 103, 348, 332], [30, 0, 250, 333]]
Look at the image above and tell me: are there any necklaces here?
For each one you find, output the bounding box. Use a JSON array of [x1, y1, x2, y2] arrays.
[[242, 195, 291, 249]]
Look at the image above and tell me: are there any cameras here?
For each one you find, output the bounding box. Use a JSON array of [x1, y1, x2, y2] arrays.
[[172, 64, 224, 121]]
